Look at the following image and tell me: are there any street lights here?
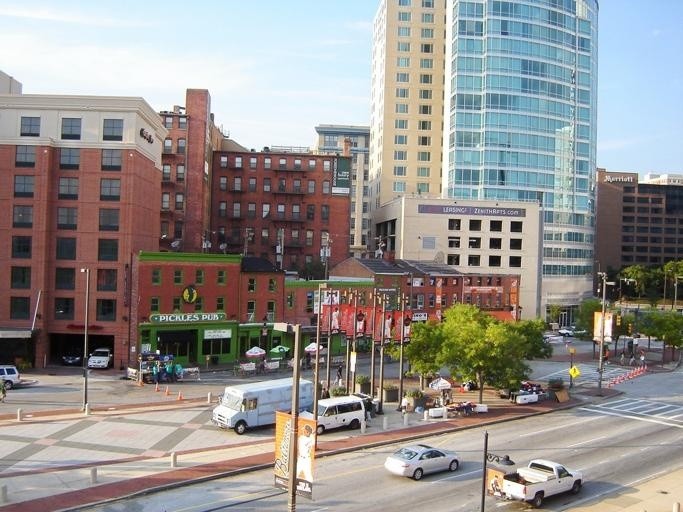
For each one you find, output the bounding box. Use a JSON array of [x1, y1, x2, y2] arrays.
[[80, 267, 91, 411], [596, 269, 683, 396]]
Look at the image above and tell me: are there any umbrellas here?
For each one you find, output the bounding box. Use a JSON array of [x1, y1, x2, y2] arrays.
[[430, 377, 451, 397], [269, 344, 291, 359], [304, 342, 324, 358], [244, 346, 268, 363]]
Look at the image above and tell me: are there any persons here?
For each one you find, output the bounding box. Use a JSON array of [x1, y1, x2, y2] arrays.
[[257, 356, 266, 375], [320, 388, 330, 400], [640, 348, 646, 367], [444, 390, 450, 405], [629, 352, 635, 367], [489, 475, 501, 494], [399, 396, 408, 417], [331, 307, 342, 330], [164, 361, 174, 383], [332, 364, 343, 385], [152, 363, 161, 384], [356, 309, 367, 336], [175, 361, 183, 379], [605, 348, 611, 365], [0, 379, 9, 404], [296, 425, 313, 485], [403, 315, 411, 342], [365, 399, 373, 422], [620, 352, 625, 365], [464, 403, 473, 416], [384, 313, 393, 344], [305, 352, 312, 369]]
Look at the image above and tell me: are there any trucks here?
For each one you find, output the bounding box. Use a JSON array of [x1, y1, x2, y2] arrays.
[[213, 376, 316, 435]]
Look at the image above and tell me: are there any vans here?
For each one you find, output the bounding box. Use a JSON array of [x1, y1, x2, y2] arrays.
[[310, 392, 370, 436]]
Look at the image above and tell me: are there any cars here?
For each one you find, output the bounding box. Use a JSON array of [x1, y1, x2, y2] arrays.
[[384, 441, 463, 481], [60, 348, 113, 370], [558, 326, 590, 338], [0, 364, 22, 390]]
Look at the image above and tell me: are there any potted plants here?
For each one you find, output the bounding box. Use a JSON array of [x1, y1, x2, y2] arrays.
[[355, 375, 427, 411]]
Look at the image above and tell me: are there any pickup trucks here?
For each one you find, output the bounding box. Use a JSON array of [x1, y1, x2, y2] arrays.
[[502, 457, 584, 508]]
